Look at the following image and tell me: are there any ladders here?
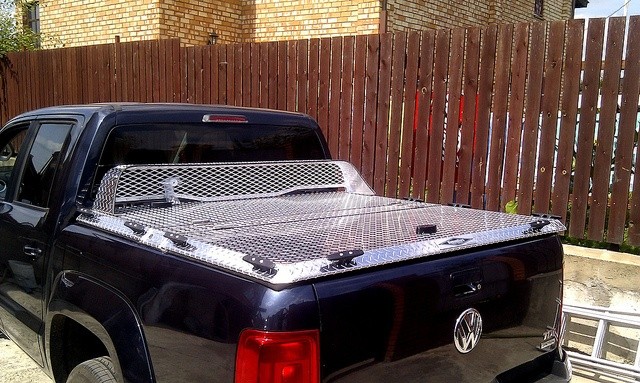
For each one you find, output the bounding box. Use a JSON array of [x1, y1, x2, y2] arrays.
[[560, 302, 640, 383]]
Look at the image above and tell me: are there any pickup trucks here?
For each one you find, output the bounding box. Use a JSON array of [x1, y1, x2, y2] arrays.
[[0, 102, 572, 382]]
